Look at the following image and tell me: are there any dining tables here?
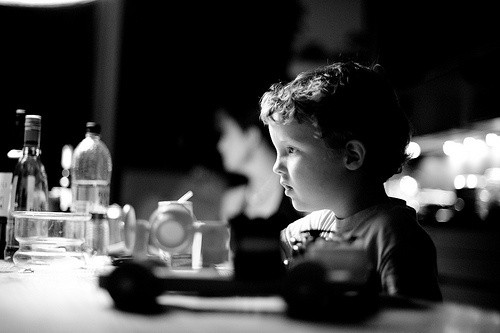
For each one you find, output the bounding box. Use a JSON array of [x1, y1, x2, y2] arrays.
[[0, 235, 499, 333]]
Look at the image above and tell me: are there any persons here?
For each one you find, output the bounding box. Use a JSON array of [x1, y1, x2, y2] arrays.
[[202, 93, 303, 254], [131, 62, 445, 304]]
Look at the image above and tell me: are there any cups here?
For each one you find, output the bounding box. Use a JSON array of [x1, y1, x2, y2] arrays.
[[12, 209, 90, 274], [191, 221, 231, 270]]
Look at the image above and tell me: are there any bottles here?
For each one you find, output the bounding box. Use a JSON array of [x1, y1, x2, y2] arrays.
[[68, 121, 113, 258], [3, 115, 50, 265], [0, 104, 27, 259]]
